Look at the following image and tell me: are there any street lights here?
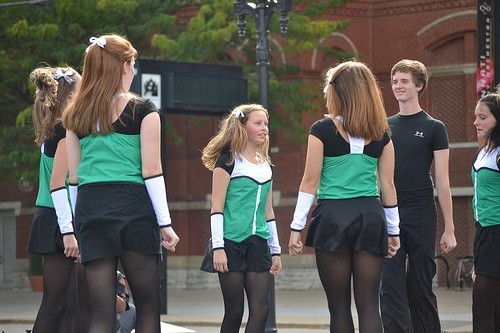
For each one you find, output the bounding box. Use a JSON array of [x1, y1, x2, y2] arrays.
[[233, 0, 291, 109]]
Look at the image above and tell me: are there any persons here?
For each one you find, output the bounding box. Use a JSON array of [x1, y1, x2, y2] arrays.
[[200, 103, 282, 332], [289, 61, 401, 333], [29, 34, 180, 333], [378, 58, 457, 333], [471, 94, 500, 333]]
